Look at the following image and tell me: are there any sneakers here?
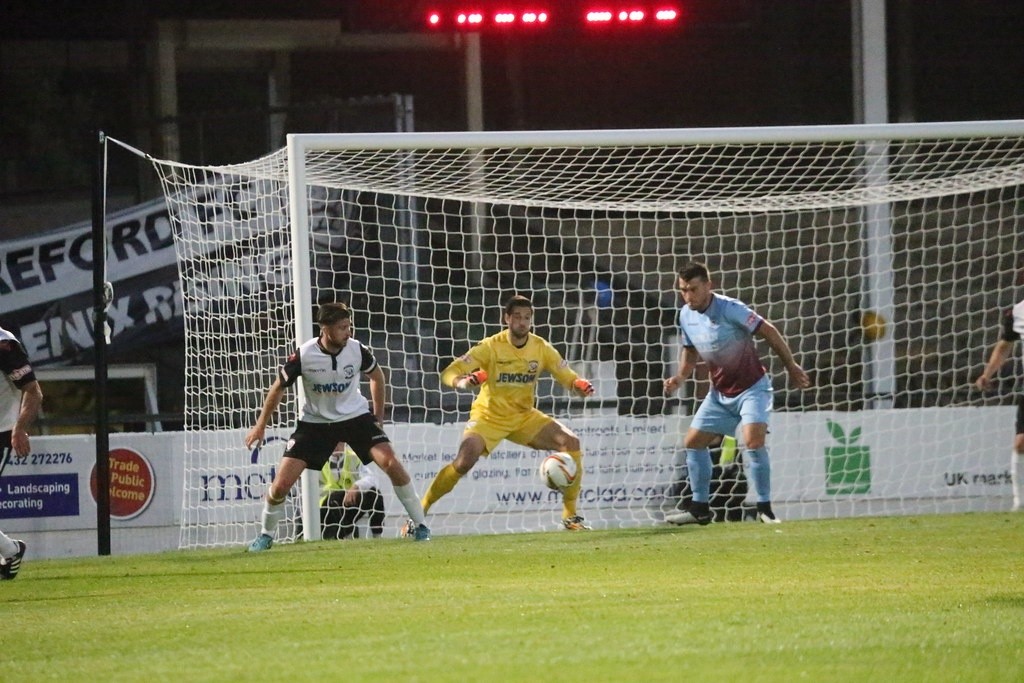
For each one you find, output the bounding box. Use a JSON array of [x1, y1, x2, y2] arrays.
[[413, 522, 430, 541], [0, 540, 25, 581], [760, 511, 780, 524], [401, 518, 417, 540], [558, 516, 592, 531], [248, 533, 273, 552], [665, 505, 710, 527]]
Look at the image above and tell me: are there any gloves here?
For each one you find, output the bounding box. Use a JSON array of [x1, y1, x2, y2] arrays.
[[574, 380, 595, 398], [467, 370, 488, 385]]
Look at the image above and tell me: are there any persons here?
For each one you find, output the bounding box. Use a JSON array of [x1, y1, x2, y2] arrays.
[[296, 441, 384, 540], [0, 326, 43, 581], [977, 300, 1024, 511], [673, 431, 748, 522], [244, 303, 431, 551], [662, 262, 809, 526], [402, 295, 594, 535]]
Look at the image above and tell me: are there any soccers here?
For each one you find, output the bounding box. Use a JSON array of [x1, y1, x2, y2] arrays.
[[540, 453, 578, 490]]
